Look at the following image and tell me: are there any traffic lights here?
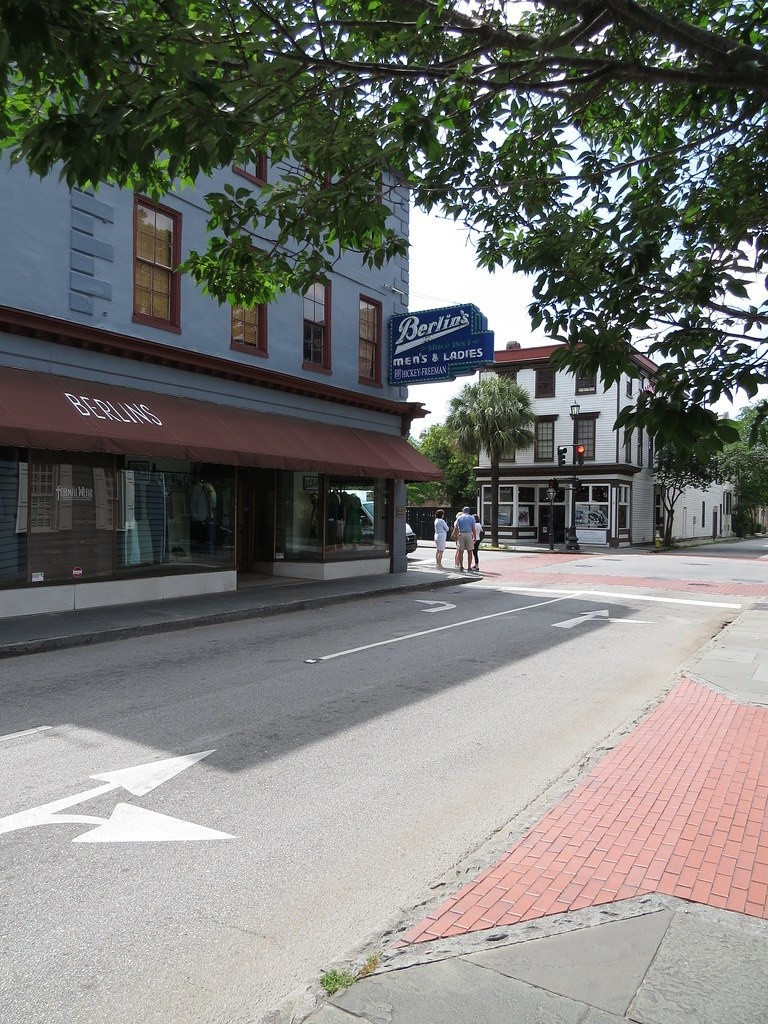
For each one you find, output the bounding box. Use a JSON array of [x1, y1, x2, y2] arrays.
[[557, 447, 568, 469], [577, 445, 586, 464]]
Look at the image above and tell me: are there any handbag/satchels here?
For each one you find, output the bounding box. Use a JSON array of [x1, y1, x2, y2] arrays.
[[450, 526, 459, 540]]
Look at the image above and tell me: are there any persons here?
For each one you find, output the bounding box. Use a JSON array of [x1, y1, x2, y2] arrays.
[[146, 471, 172, 562], [186, 475, 216, 553], [328, 489, 362, 546], [433, 509, 449, 568], [451, 506, 485, 571]]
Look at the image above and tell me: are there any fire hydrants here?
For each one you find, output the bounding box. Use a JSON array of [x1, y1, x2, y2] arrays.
[[655, 533, 661, 549]]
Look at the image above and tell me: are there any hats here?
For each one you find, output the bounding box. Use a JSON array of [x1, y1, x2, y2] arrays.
[[462, 506, 470, 511]]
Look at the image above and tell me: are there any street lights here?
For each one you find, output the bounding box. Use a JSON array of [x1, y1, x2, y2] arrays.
[[566, 399, 583, 551], [547, 487, 556, 550]]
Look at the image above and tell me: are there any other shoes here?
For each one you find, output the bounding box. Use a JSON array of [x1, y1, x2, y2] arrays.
[[467, 568, 473, 572], [472, 566, 480, 572], [459, 567, 465, 571], [456, 566, 460, 568], [436, 565, 446, 570], [454, 565, 458, 567]]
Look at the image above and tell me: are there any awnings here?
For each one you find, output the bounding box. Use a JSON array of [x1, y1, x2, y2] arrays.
[[0, 366, 446, 483]]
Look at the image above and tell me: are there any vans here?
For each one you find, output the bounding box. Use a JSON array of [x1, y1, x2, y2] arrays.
[[359, 501, 417, 556]]
